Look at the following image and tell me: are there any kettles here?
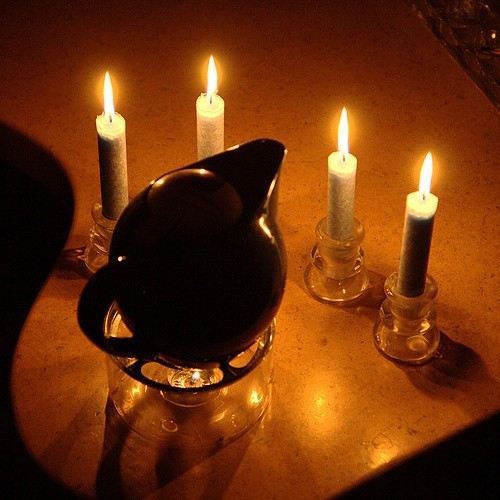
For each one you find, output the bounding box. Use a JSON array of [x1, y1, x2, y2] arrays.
[[75, 138, 290, 371]]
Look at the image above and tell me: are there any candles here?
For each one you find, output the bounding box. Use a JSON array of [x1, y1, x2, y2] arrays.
[[323, 104, 365, 245], [155, 366, 220, 409], [84, 69, 133, 223], [190, 52, 227, 163], [393, 148, 440, 299]]
